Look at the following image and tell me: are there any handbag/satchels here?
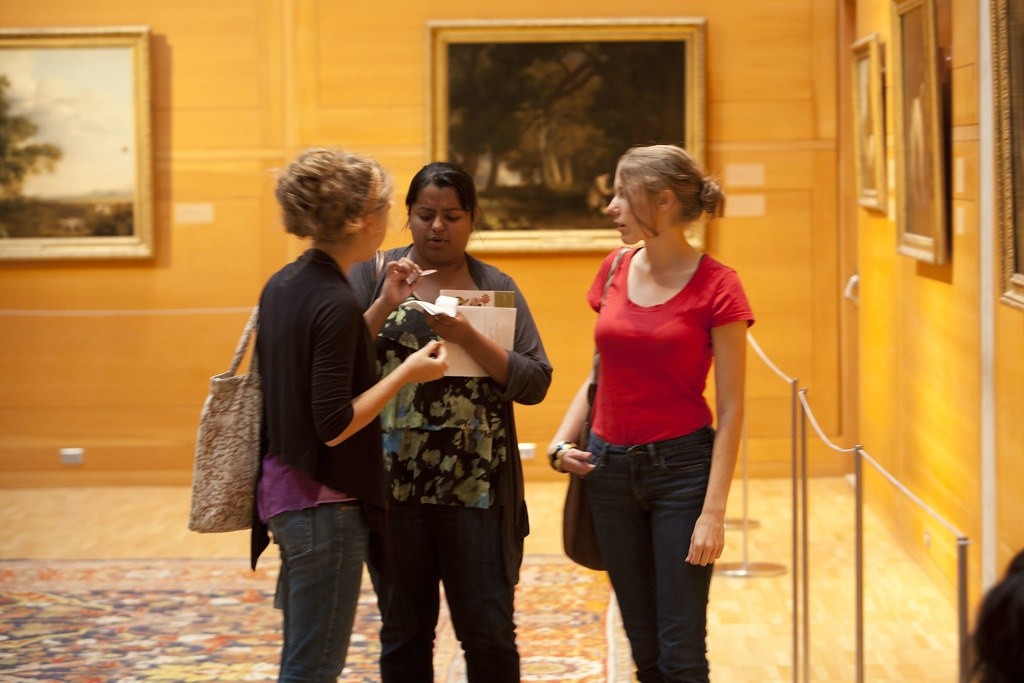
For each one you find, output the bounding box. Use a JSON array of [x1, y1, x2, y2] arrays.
[[561, 423, 607, 572], [188, 305, 261, 532]]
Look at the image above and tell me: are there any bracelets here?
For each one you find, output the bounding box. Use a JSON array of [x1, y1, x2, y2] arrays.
[[551, 441, 577, 472]]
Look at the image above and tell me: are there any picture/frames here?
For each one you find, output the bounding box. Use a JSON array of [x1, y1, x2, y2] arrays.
[[989, 0, 1024, 311], [893, 1, 948, 268], [0, 25, 154, 261], [424, 18, 706, 256], [849, 33, 887, 213]]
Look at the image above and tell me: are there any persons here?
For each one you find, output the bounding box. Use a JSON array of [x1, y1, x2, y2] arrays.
[[248, 148, 447, 683], [548, 146, 755, 683], [343, 161, 554, 683]]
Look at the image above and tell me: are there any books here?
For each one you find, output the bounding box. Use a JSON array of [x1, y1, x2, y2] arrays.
[[438, 288, 516, 378]]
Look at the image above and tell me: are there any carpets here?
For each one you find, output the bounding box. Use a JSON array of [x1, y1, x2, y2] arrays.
[[0, 558, 629, 683]]
